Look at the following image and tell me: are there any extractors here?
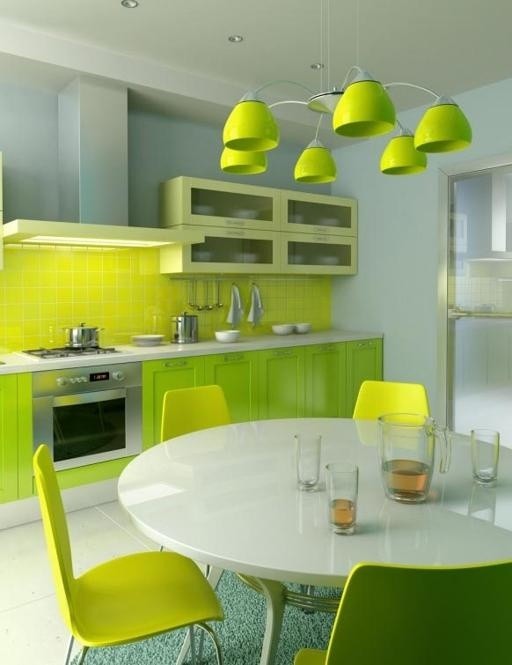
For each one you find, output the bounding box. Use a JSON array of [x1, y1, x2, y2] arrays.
[[1, 221, 205, 250]]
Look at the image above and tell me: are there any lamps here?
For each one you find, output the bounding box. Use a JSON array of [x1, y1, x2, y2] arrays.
[[217, 0, 475, 187]]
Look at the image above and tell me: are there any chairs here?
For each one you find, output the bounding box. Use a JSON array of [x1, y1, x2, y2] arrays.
[[291, 559, 512, 664], [161, 385, 232, 446], [32, 444, 225, 665], [351, 378, 430, 426]]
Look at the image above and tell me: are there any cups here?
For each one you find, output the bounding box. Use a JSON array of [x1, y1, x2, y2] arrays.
[[294, 430, 322, 491], [325, 461, 359, 535], [470, 428, 500, 485]]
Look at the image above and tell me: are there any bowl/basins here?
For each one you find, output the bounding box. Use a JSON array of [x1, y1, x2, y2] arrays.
[[295, 323, 312, 333], [215, 330, 241, 343], [271, 325, 295, 336]]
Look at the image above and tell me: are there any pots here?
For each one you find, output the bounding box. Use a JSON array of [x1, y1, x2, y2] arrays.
[[170, 315, 198, 344], [63, 322, 105, 349]]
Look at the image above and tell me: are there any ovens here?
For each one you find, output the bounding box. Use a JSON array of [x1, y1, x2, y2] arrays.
[[31, 361, 143, 476]]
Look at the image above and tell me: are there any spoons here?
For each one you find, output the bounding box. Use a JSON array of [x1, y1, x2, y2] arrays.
[[187, 279, 223, 311]]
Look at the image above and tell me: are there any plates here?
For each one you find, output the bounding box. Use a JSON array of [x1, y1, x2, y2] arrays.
[[291, 215, 306, 223], [238, 209, 258, 218], [316, 256, 339, 264], [194, 207, 215, 214], [289, 255, 306, 263], [321, 217, 340, 226], [131, 334, 165, 348], [193, 252, 212, 261], [237, 252, 256, 262]]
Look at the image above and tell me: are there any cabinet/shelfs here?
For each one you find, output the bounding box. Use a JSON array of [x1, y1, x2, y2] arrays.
[[157, 174, 358, 277], [204, 350, 257, 423], [305, 342, 345, 419], [347, 338, 384, 419], [141, 355, 204, 454], [258, 345, 305, 419], [0, 370, 34, 506]]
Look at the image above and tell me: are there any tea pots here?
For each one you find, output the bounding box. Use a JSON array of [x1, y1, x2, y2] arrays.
[[376, 412, 452, 504]]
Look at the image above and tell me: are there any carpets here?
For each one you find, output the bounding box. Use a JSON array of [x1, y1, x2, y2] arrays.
[[68, 564, 346, 665]]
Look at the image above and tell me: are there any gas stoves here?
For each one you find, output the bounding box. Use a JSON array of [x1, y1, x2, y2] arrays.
[[20, 345, 119, 360]]
